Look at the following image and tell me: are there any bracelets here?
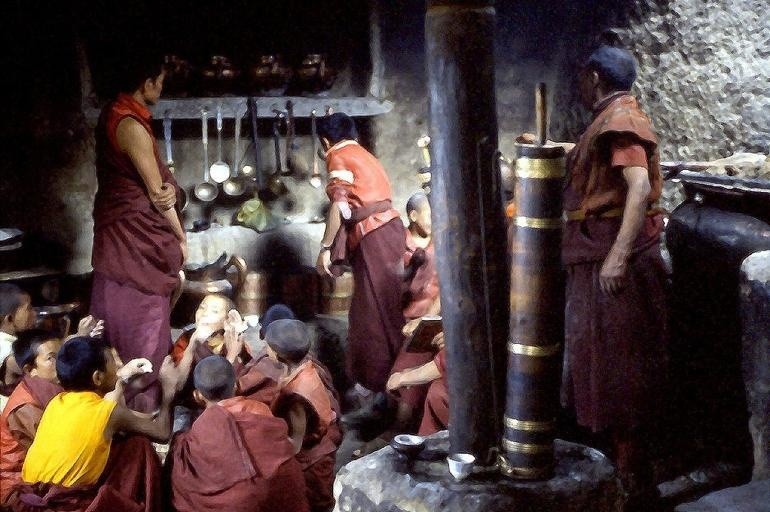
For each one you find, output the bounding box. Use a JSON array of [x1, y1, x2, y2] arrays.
[[320, 246, 332, 251]]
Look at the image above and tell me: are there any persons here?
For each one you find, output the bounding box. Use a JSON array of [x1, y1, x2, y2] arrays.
[[0, 281, 345, 511], [541, 46, 673, 512], [315, 112, 408, 425], [384, 193, 517, 437], [87, 38, 188, 414]]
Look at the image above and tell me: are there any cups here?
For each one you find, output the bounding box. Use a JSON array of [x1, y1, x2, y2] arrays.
[[447, 454, 476, 479]]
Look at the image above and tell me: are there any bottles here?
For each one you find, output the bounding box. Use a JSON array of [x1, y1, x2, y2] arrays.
[[164, 52, 333, 97]]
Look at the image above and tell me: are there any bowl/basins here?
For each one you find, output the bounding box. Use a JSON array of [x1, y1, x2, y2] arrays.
[[193, 220, 209, 231], [390, 432, 426, 456]]
